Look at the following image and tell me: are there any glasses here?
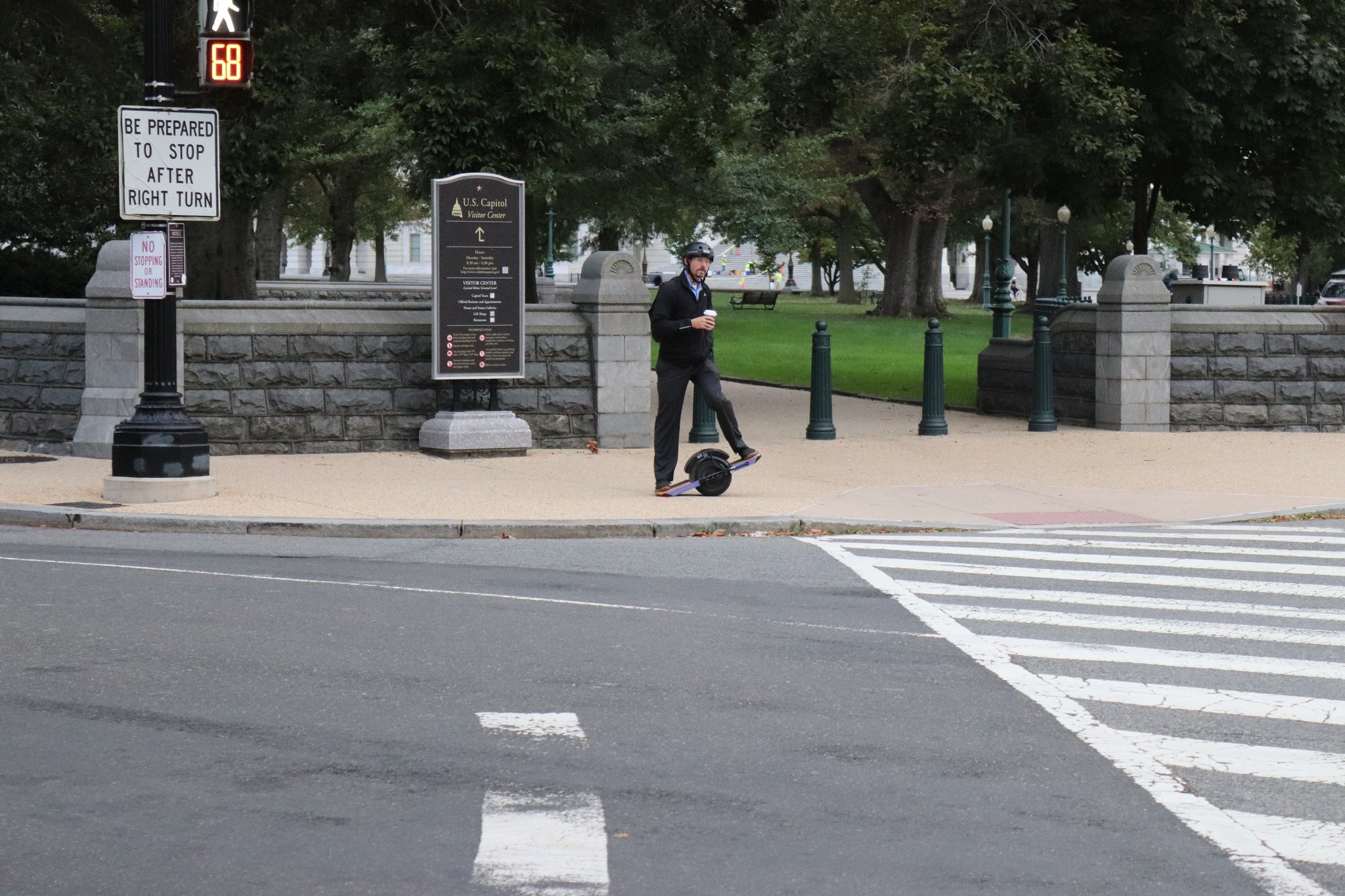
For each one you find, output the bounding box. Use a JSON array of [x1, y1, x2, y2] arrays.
[[690, 257, 710, 264]]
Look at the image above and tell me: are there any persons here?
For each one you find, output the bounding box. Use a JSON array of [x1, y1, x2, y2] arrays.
[[775, 269, 785, 289], [750, 260, 757, 274], [770, 273, 776, 289], [1011, 277, 1021, 300], [1265, 292, 1317, 304], [721, 255, 727, 271], [745, 261, 751, 276], [651, 242, 758, 496]]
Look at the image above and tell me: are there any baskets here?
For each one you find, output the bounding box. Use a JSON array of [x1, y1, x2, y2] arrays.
[[1022, 289, 1027, 293]]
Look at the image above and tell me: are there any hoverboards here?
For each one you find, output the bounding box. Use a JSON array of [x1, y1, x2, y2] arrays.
[[654, 448, 761, 496]]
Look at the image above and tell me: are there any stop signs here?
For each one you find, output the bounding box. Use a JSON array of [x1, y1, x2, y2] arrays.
[[130, 231, 167, 300], [119, 105, 220, 219]]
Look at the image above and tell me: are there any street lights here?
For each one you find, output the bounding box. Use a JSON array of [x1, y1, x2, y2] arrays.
[[544, 184, 558, 281], [1207, 224, 1217, 280], [1057, 203, 1071, 299], [1126, 240, 1134, 255], [980, 215, 992, 311]]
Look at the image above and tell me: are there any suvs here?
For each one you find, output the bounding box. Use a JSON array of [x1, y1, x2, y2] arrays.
[[1313, 279, 1345, 306]]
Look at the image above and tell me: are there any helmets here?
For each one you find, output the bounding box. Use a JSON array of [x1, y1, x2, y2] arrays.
[[1012, 277, 1017, 280], [748, 262, 751, 264], [754, 261, 757, 263], [722, 255, 726, 258], [680, 242, 714, 262]]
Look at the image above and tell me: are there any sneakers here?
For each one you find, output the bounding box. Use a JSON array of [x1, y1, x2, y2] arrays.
[[655, 480, 672, 492], [734, 447, 757, 460]]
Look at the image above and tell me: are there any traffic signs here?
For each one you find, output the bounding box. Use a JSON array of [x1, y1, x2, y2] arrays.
[[432, 172, 526, 379]]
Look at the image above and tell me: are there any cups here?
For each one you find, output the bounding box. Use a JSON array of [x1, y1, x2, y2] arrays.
[[704, 310, 717, 331]]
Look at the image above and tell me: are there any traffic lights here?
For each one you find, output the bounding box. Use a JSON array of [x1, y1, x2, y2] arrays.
[[197, 0, 251, 87]]
